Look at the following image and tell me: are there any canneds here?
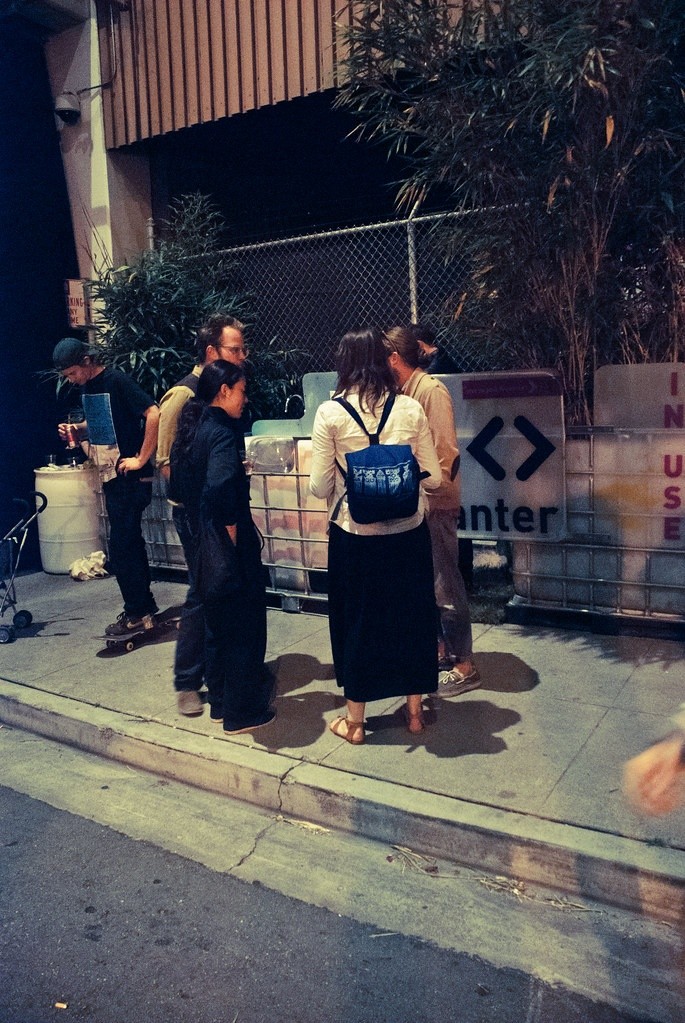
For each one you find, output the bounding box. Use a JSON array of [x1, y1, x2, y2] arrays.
[[65, 425, 80, 448]]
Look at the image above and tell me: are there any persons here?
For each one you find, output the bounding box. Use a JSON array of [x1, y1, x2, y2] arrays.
[[166, 359, 278, 733], [306, 328, 443, 745], [155, 310, 246, 715], [52, 337, 161, 635], [379, 325, 483, 700]]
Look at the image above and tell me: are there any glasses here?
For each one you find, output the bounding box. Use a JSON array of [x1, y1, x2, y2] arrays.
[[379, 329, 399, 354], [212, 345, 249, 359]]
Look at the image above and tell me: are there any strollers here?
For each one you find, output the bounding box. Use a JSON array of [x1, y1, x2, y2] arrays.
[[0, 493, 47, 644]]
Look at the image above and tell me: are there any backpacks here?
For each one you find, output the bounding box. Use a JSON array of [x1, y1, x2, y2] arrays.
[[332, 391, 421, 525]]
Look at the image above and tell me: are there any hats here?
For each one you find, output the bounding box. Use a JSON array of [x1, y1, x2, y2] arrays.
[[53, 338, 98, 371]]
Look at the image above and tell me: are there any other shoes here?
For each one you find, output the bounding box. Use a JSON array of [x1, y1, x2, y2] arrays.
[[210, 704, 224, 722], [223, 709, 277, 735], [178, 691, 204, 714]]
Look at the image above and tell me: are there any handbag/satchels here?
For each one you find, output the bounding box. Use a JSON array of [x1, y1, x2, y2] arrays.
[[69, 551, 110, 580]]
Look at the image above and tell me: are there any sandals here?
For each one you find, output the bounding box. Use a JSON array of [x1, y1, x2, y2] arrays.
[[330, 716, 366, 745], [401, 703, 426, 735]]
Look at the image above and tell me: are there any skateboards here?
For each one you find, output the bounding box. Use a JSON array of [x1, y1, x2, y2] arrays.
[[93, 605, 182, 651]]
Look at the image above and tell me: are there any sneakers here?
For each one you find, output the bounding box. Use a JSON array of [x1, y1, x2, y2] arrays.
[[438, 657, 454, 670], [105, 611, 149, 636], [428, 666, 482, 699]]
